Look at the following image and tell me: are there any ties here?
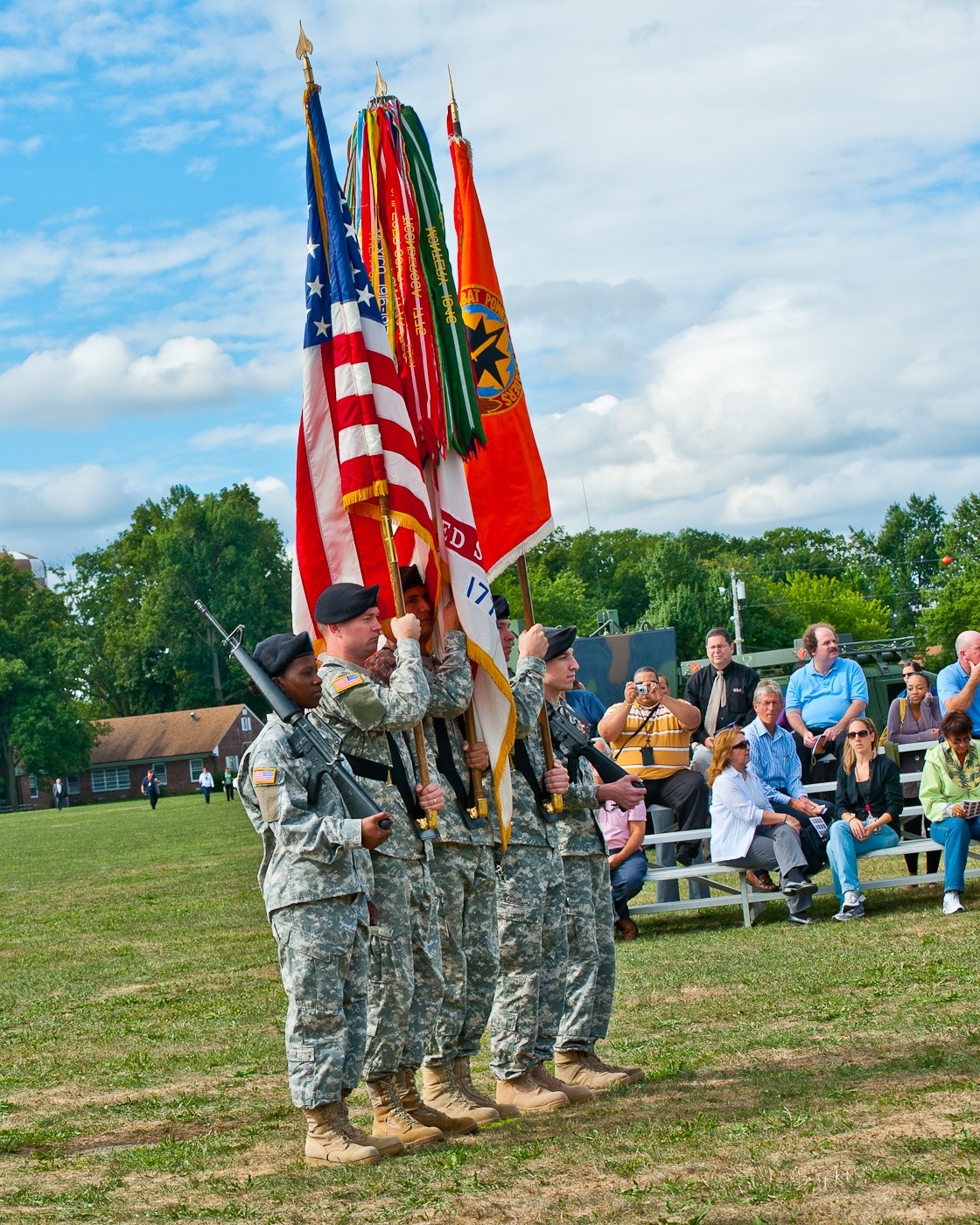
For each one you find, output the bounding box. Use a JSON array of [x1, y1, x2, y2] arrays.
[[704, 670, 726, 737]]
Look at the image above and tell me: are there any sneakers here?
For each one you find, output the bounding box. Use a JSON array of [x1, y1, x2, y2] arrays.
[[832, 901, 867, 921], [942, 890, 967, 914], [839, 893, 867, 914]]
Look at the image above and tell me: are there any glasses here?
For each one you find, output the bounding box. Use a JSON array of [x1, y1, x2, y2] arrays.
[[732, 739, 750, 749], [847, 730, 871, 738], [948, 735, 971, 745], [901, 672, 915, 677]]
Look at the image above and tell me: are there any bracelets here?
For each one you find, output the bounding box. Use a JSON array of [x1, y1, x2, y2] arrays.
[[783, 813, 788, 822], [848, 817, 856, 823]]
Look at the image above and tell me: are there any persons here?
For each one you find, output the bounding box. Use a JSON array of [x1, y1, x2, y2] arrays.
[[597, 665, 706, 862], [559, 679, 646, 942], [515, 620, 646, 1090], [883, 631, 980, 914], [238, 630, 404, 1171], [363, 563, 521, 1127], [826, 716, 903, 923], [449, 594, 590, 1111], [52, 764, 236, 811], [303, 584, 477, 1147], [655, 674, 711, 900], [684, 621, 868, 928]]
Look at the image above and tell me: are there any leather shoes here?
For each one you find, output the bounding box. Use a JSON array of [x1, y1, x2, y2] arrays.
[[788, 913, 823, 924], [783, 879, 818, 897], [747, 871, 781, 892]]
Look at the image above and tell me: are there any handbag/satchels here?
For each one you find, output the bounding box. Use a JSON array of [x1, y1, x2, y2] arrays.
[[879, 698, 905, 746]]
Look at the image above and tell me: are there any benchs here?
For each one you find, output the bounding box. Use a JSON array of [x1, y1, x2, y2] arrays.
[[629, 740, 980, 928]]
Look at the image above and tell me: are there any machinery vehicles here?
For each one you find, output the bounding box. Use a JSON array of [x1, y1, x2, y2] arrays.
[[505, 610, 939, 744]]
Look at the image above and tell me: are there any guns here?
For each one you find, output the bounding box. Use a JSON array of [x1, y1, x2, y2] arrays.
[[508, 667, 649, 813], [196, 598, 392, 839]]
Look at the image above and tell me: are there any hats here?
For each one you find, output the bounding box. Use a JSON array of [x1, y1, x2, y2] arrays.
[[541, 626, 578, 662], [251, 632, 315, 679], [492, 595, 511, 620], [313, 582, 380, 625], [398, 564, 424, 590]]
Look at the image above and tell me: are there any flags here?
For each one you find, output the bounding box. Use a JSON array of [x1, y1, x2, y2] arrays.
[[288, 85, 554, 851]]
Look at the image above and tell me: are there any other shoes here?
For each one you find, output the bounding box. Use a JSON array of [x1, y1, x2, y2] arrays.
[[617, 918, 636, 940], [907, 884, 918, 890], [930, 883, 940, 889]]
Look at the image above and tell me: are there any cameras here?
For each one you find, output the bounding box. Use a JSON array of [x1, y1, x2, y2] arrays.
[[635, 684, 648, 694]]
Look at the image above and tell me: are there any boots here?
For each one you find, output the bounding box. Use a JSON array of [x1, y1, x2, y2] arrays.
[[422, 1055, 522, 1127], [302, 1097, 404, 1167], [496, 1062, 593, 1113], [552, 1050, 646, 1093], [366, 1067, 479, 1148]]
